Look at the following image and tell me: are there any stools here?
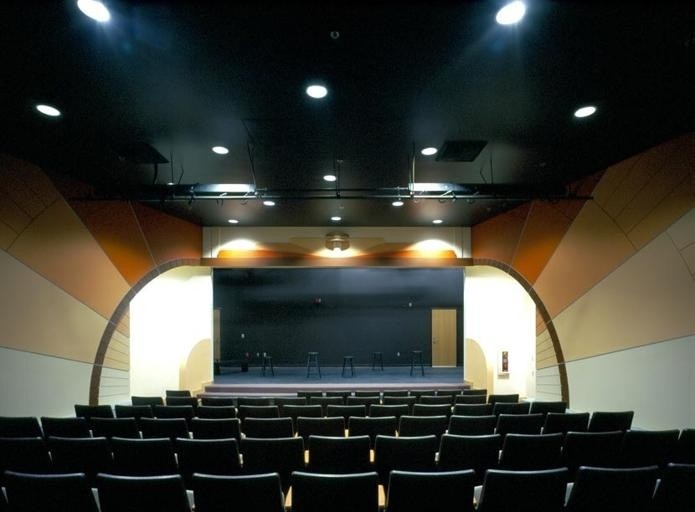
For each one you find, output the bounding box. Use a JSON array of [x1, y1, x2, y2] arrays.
[[261, 354, 275, 377], [372, 352, 384, 372], [342, 356, 354, 377], [410, 351, 425, 376], [307, 352, 322, 378]]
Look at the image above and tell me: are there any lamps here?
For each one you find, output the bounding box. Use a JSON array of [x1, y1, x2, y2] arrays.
[[325, 232, 350, 252], [336, 152, 344, 198]]
